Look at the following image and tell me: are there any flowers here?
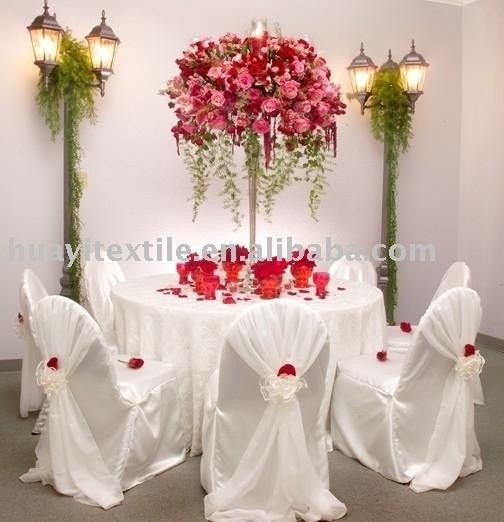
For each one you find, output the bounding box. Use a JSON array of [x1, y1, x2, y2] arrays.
[[156, 11, 348, 233]]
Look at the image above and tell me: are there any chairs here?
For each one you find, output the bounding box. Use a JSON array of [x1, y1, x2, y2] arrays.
[[18, 256, 485, 522]]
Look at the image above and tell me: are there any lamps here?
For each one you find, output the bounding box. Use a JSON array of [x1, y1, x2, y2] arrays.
[[25, 0, 121, 96], [345, 38, 432, 116]]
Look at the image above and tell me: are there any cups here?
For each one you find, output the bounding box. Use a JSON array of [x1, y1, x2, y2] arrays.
[[201, 275, 220, 301], [176, 263, 190, 285], [313, 272, 330, 296]]
